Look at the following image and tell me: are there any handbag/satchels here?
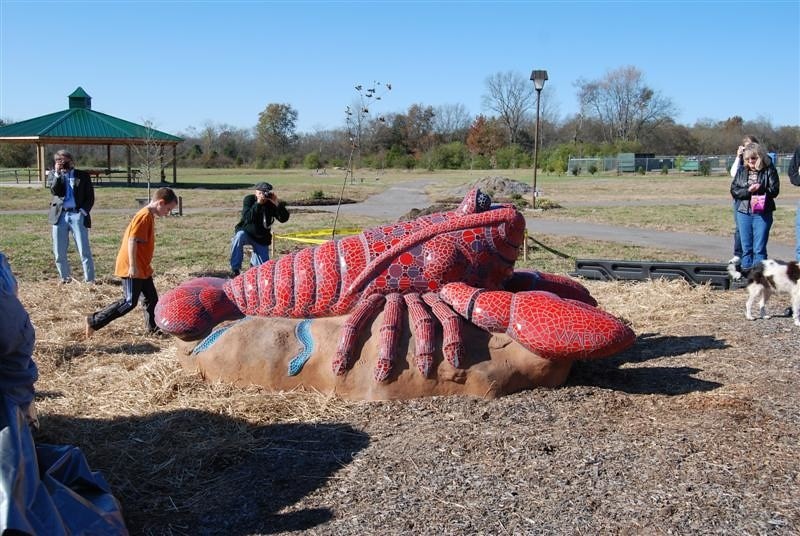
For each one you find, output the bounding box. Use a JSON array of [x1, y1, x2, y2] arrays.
[[751, 193, 767, 214]]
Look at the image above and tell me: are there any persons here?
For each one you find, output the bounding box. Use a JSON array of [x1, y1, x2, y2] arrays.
[[48, 149, 95, 286], [230, 182, 289, 279], [0, 253, 41, 433], [788, 144, 800, 265], [84, 187, 178, 339], [730, 134, 780, 269]]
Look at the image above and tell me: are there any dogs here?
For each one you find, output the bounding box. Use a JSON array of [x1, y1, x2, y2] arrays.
[[728, 259, 800, 327]]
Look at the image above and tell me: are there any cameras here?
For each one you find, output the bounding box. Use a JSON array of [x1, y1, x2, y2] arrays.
[[262, 192, 272, 199], [60, 161, 70, 169]]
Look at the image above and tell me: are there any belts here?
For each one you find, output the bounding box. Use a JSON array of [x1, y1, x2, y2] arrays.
[[62, 207, 78, 212]]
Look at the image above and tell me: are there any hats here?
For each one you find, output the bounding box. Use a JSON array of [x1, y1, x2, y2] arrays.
[[257, 182, 273, 191]]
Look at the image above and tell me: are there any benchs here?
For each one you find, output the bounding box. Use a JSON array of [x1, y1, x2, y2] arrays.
[[10, 168, 140, 189], [136, 195, 172, 215]]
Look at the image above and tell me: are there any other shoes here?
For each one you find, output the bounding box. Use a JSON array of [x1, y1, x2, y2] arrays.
[[729, 255, 741, 265], [146, 328, 169, 339], [227, 271, 240, 278]]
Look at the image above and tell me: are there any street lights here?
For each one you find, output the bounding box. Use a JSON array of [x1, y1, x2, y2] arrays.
[[529, 68, 549, 210]]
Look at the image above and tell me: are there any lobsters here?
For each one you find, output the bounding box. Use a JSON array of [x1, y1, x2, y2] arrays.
[[154, 188, 625, 380]]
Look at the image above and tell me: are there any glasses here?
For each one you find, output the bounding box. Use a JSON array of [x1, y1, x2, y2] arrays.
[[745, 156, 757, 161]]
